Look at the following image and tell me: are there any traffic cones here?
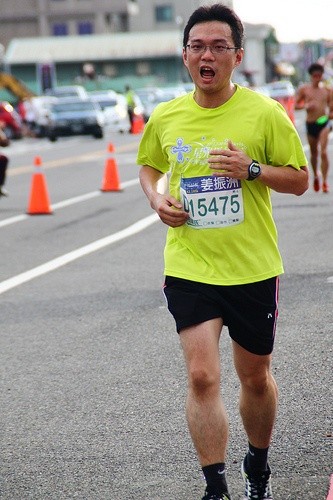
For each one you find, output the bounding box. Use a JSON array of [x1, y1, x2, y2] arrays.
[[23, 156, 55, 216], [131, 107, 145, 136], [99, 141, 125, 194]]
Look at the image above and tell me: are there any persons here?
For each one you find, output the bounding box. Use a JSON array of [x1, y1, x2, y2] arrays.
[[136, 4, 308, 500], [295, 64, 333, 193], [125, 86, 136, 133]]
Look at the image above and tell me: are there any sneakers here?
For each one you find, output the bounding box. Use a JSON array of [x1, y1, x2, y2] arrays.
[[314, 177, 319, 192], [321, 183, 328, 193], [202, 492, 232, 500], [241, 454, 274, 500]]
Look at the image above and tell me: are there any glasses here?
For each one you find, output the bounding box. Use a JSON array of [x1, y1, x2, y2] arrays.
[[311, 74, 321, 79], [185, 41, 240, 54]]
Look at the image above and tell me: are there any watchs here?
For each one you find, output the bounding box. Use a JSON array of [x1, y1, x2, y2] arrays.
[[246, 159, 262, 181]]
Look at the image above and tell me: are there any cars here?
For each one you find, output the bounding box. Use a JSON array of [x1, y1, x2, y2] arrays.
[[267, 80, 296, 101], [21, 81, 194, 143]]
